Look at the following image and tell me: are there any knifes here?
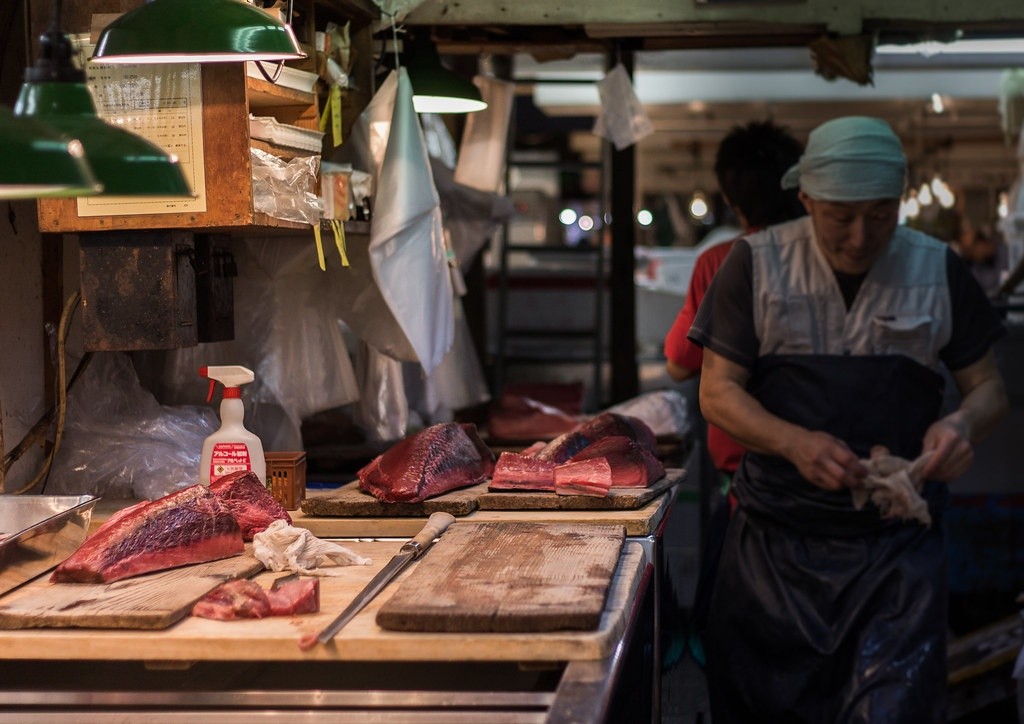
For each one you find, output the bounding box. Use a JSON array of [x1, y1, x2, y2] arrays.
[[316, 510, 454, 645]]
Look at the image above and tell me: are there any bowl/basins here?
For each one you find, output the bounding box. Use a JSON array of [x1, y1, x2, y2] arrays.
[[0, 495, 102, 597]]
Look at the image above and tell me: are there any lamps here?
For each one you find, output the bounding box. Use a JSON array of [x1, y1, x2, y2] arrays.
[[86, 1, 308, 64], [399, 25, 488, 115], [0, 0, 199, 200]]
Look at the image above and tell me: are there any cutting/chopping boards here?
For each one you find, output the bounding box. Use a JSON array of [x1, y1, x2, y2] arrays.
[[301, 456, 685, 515], [376, 521, 627, 630], [0, 531, 262, 630]]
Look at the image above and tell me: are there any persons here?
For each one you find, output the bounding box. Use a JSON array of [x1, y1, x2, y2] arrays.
[[664, 121, 805, 511], [687, 113, 1010, 724]]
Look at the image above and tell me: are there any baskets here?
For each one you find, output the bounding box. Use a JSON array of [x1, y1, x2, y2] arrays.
[[261, 450, 307, 510]]
